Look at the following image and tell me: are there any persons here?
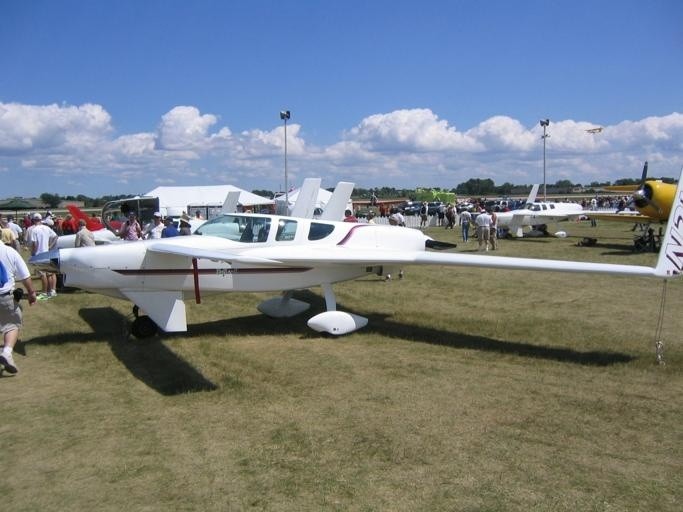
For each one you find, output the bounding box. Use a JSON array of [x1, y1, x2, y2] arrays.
[[105, 208, 204, 241], [0, 239, 37, 375], [1, 209, 62, 301], [379, 199, 457, 230], [61, 212, 100, 247], [458, 195, 527, 251], [562, 195, 647, 232], [343, 210, 374, 223]]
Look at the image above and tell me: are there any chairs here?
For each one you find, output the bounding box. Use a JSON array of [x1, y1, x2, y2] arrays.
[[238, 220, 286, 242]]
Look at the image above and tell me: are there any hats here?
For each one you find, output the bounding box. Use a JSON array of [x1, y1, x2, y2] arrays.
[[77, 219, 86, 224], [195, 209, 200, 214], [423, 201, 429, 206], [480, 209, 485, 212], [162, 216, 173, 221], [154, 211, 163, 217], [179, 215, 191, 224], [32, 212, 42, 220], [127, 211, 136, 216]]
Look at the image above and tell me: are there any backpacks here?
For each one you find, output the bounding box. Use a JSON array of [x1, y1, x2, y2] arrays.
[[420, 206, 427, 213]]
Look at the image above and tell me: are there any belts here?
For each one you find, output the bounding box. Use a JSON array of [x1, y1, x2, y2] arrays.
[[1, 292, 10, 295]]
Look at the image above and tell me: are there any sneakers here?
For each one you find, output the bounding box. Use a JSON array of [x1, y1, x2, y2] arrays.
[[463, 240, 499, 251], [37, 289, 57, 300], [0, 352, 18, 373], [384, 272, 404, 279]]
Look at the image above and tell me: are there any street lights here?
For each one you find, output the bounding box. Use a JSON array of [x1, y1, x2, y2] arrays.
[[538, 118, 550, 203], [277, 107, 291, 215]]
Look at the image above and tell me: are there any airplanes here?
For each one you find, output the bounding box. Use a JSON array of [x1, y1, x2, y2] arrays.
[[25, 177, 683, 339], [584, 161, 678, 250], [492, 183, 641, 238], [584, 127, 604, 135]]
